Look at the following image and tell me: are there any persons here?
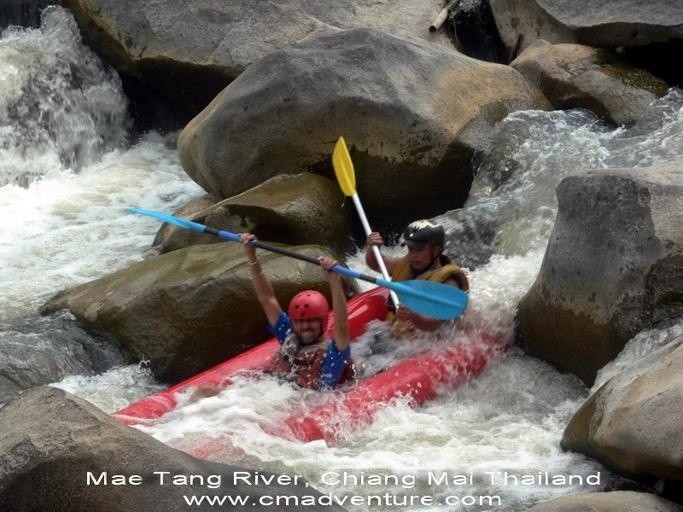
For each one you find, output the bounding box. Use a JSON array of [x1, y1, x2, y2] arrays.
[[238, 230, 350, 393], [364, 219, 470, 340]]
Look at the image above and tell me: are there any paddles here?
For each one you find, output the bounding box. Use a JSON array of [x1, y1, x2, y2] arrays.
[[332, 136, 400, 311], [125, 208, 468, 320]]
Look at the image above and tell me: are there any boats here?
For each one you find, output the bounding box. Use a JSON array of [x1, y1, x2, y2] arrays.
[[108, 285, 507, 463]]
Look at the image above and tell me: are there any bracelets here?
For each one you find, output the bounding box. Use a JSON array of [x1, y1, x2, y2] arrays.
[[246, 258, 260, 267]]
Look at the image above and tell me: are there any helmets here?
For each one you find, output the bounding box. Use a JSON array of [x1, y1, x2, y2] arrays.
[[403, 219, 445, 246], [289, 290, 329, 332]]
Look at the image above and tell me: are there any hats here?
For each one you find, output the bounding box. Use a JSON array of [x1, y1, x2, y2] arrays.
[[401, 240, 424, 250]]
